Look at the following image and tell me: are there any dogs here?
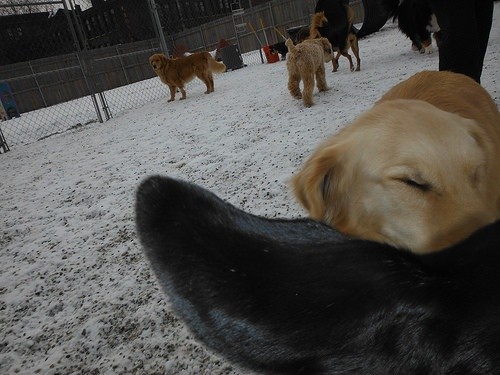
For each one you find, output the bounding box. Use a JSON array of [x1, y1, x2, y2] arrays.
[[135, 174, 500, 375], [310, 0, 361, 72], [285, 38, 334, 107], [356, 0, 493, 83], [147, 51, 227, 103], [290, 70, 500, 254]]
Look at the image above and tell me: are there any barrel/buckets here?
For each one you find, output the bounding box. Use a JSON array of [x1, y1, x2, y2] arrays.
[[263, 45, 279, 64], [219, 43, 244, 69]]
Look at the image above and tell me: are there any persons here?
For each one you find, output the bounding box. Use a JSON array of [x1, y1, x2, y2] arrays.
[[431, 0, 494, 85]]
